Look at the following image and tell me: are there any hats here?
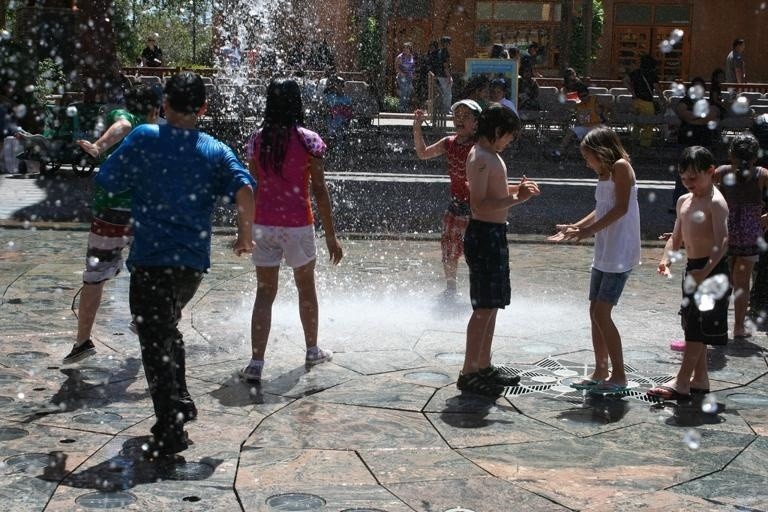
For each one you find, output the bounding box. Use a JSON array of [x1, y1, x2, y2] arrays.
[[450, 98, 483, 113]]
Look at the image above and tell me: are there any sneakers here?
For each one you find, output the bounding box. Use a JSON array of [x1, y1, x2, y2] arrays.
[[180, 395, 199, 421], [127, 321, 138, 337], [457, 371, 504, 397], [306, 345, 335, 366], [238, 362, 265, 383], [483, 367, 522, 385], [61, 340, 97, 364], [142, 431, 189, 459]]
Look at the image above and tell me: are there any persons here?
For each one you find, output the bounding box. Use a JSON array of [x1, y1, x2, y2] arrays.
[[100, 61, 130, 104], [1, 102, 23, 174], [412, 100, 539, 394], [219, 30, 352, 153], [63, 85, 163, 366], [394, 36, 587, 112], [92, 69, 255, 451], [545, 128, 642, 392], [237, 77, 342, 380], [623, 54, 666, 155], [647, 146, 730, 400], [676, 37, 767, 337], [142, 34, 163, 76]]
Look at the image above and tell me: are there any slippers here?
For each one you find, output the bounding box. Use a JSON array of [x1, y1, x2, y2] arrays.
[[592, 382, 631, 394], [647, 383, 691, 401], [569, 376, 599, 389], [688, 381, 711, 393]]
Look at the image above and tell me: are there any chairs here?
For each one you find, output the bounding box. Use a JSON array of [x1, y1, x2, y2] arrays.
[[529, 82, 768, 147], [125, 74, 380, 134]]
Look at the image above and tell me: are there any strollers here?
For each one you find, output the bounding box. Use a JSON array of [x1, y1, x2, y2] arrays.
[[17, 76, 115, 180]]
